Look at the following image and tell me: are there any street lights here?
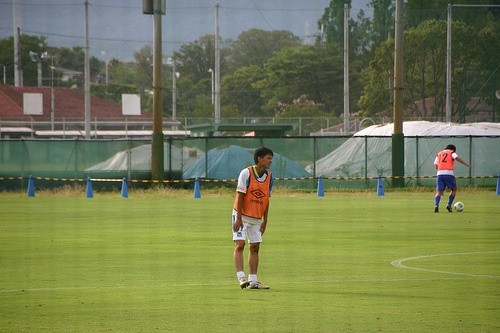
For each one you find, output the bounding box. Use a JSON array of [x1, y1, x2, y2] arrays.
[[208, 69, 215, 117], [105, 58, 112, 83]]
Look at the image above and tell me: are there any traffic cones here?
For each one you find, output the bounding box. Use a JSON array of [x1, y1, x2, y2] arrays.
[[496, 172, 500, 196], [317, 176, 324, 197], [193, 176, 201, 198], [26, 174, 35, 197], [86, 177, 93, 198], [376, 174, 384, 196], [121, 177, 128, 198]]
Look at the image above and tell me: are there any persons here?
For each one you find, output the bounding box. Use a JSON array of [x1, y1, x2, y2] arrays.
[[231, 147, 274, 289], [432, 143, 471, 213]]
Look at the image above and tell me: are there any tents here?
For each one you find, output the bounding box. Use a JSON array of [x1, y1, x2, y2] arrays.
[[182, 143, 316, 183], [300, 120, 500, 181], [84, 143, 206, 175]]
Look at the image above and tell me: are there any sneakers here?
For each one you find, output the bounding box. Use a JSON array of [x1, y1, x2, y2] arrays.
[[435, 206, 439, 213], [248, 280, 269, 289], [238, 276, 250, 289], [446, 205, 453, 212]]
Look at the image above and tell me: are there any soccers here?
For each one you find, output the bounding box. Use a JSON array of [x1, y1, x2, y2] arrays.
[[453, 201, 464, 212]]
